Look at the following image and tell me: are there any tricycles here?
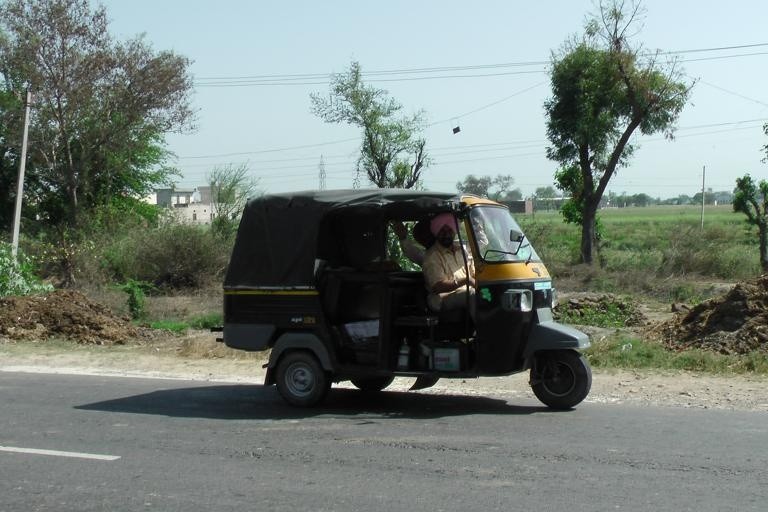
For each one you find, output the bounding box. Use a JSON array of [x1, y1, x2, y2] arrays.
[[209, 189, 590, 410]]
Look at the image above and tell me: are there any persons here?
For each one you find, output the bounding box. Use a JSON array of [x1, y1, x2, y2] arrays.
[[388, 217, 434, 267], [422, 214, 477, 331]]
